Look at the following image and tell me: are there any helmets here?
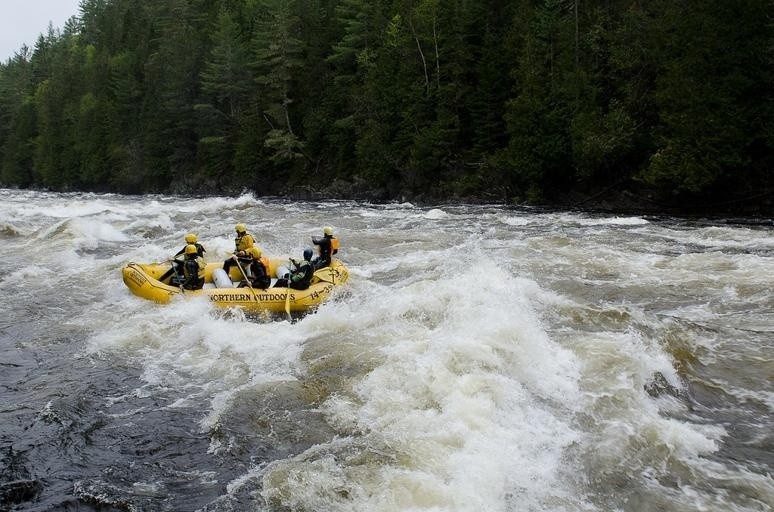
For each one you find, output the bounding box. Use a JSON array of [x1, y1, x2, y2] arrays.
[[303, 247, 313, 259], [185, 234, 198, 243], [250, 248, 261, 259], [324, 227, 333, 236], [235, 224, 246, 233], [183, 245, 198, 254]]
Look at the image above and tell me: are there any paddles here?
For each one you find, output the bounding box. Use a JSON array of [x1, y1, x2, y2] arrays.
[[224, 249, 240, 257], [285, 260, 292, 315], [236, 259, 269, 320]]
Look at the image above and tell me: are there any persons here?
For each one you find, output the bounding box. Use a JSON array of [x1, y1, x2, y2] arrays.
[[223, 224, 256, 277], [169, 244, 206, 290], [157, 234, 206, 281], [312, 227, 339, 271], [273, 249, 315, 290], [232, 247, 271, 288]]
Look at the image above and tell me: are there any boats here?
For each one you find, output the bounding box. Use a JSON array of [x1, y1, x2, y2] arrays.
[[122, 258, 350, 313]]
